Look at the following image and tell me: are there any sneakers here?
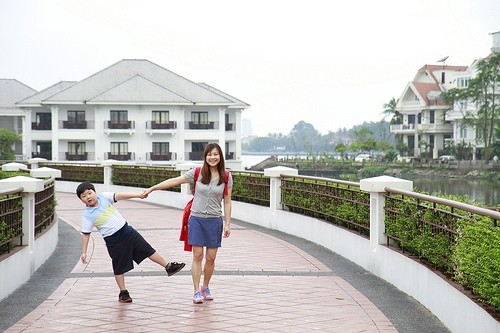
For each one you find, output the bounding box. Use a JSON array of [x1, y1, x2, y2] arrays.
[[193, 291, 204, 303], [118, 291, 132, 302], [201, 286, 213, 301], [166, 262, 186, 277]]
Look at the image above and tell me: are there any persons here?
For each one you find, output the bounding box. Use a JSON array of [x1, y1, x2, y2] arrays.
[[143, 143, 233, 304], [74, 182, 186, 303]]
